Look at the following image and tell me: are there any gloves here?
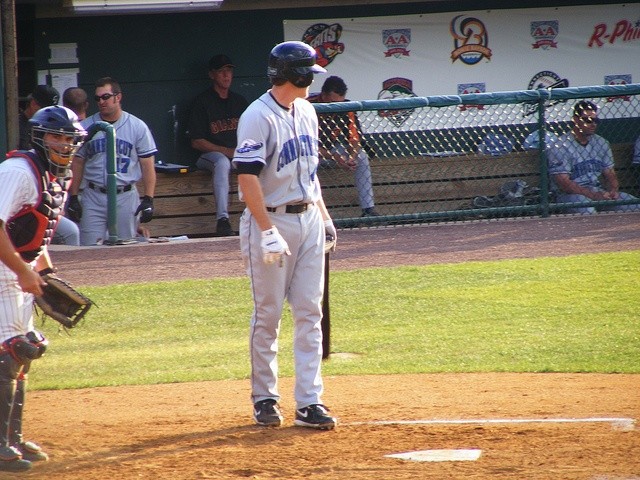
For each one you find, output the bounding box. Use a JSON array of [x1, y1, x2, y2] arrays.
[[260, 225, 292, 264], [326, 221, 337, 251], [67, 195, 82, 222], [134, 195, 153, 222]]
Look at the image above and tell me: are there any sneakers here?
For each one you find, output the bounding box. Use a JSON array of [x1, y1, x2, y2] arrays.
[[358, 212, 387, 227], [216, 217, 236, 236], [16, 443, 50, 461], [0, 459, 32, 471], [253, 399, 283, 426], [295, 405, 334, 430]]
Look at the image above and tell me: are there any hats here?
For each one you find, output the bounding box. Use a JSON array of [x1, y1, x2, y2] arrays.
[[32, 84, 59, 107], [209, 54, 237, 71]]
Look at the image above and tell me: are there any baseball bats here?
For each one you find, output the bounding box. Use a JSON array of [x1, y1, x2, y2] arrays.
[[321, 235, 333, 359]]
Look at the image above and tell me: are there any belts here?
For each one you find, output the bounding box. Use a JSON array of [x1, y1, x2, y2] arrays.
[[89, 183, 130, 193], [266, 203, 307, 213]]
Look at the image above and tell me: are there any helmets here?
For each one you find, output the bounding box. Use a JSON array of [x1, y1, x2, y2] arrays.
[[28, 105, 87, 177], [267, 41, 328, 88]]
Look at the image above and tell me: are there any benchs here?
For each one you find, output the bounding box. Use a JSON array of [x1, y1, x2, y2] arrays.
[[136, 140, 639, 237]]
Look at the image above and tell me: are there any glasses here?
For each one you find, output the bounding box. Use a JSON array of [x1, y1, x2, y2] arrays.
[[574, 116, 600, 125], [94, 93, 118, 100]]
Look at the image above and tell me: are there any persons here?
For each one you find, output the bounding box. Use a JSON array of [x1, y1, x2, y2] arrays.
[[62, 87, 89, 122], [547, 100, 640, 214], [66, 79, 157, 245], [18, 84, 59, 148], [184, 54, 242, 235], [231, 41, 337, 428], [300, 76, 378, 217], [0, 104, 89, 472]]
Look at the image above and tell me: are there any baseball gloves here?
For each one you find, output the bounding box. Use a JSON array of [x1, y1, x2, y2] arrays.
[[34, 275, 92, 328]]
